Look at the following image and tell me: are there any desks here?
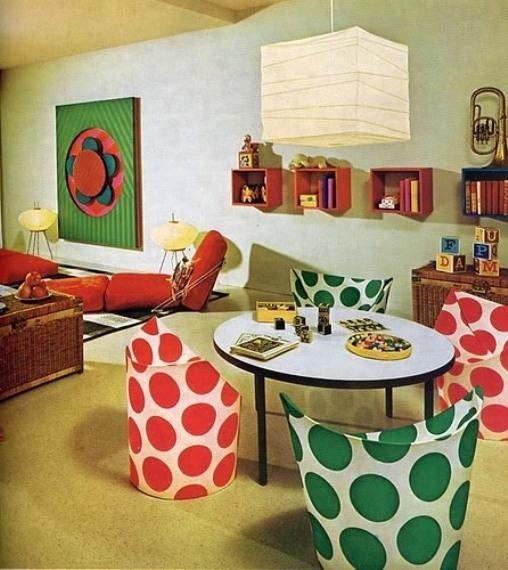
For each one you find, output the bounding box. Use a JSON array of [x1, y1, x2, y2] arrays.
[[212, 307, 455, 486], [0, 289, 83, 400]]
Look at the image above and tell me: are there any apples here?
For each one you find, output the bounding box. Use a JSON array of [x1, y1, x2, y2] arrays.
[[18, 271, 48, 298]]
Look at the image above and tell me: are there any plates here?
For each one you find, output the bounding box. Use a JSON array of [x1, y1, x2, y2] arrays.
[[13, 289, 53, 301]]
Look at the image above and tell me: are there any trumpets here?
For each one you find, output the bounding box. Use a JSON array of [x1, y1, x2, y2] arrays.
[[469, 85, 505, 171]]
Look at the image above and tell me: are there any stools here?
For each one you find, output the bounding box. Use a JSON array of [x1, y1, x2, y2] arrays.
[[279, 385, 483, 569], [288, 268, 393, 314], [434, 288, 508, 442], [125, 316, 241, 499]]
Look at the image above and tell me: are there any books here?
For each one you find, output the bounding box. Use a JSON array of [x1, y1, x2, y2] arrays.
[[470, 181, 476, 213], [337, 318, 389, 334], [504, 181, 508, 215], [465, 181, 470, 213], [300, 195, 316, 201], [400, 179, 405, 211], [481, 181, 486, 215], [405, 178, 411, 212], [492, 181, 499, 213], [301, 201, 317, 208], [318, 178, 323, 209], [411, 179, 418, 213], [328, 178, 334, 208], [486, 180, 492, 215], [499, 180, 504, 214], [323, 174, 328, 208], [477, 181, 481, 213]]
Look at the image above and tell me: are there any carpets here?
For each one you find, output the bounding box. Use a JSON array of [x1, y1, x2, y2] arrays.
[[2, 255, 229, 343]]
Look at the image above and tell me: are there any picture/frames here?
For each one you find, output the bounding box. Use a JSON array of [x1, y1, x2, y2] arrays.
[[55, 97, 144, 248], [229, 333, 260, 353]]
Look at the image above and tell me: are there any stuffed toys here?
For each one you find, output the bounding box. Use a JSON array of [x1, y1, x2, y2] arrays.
[[261, 178, 268, 204], [288, 153, 352, 168], [380, 194, 398, 209], [239, 134, 259, 168], [241, 184, 259, 203]]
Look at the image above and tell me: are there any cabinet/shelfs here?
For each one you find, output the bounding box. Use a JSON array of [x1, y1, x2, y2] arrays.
[[231, 167, 282, 208], [461, 168, 508, 217], [294, 168, 351, 211], [412, 259, 508, 333], [369, 167, 433, 215]]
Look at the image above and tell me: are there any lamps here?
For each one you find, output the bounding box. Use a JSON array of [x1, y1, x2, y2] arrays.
[[261, 0, 410, 147], [19, 201, 56, 261], [152, 213, 196, 273]]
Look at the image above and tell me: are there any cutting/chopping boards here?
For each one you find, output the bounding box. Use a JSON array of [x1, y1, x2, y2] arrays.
[[344, 332, 412, 360]]
